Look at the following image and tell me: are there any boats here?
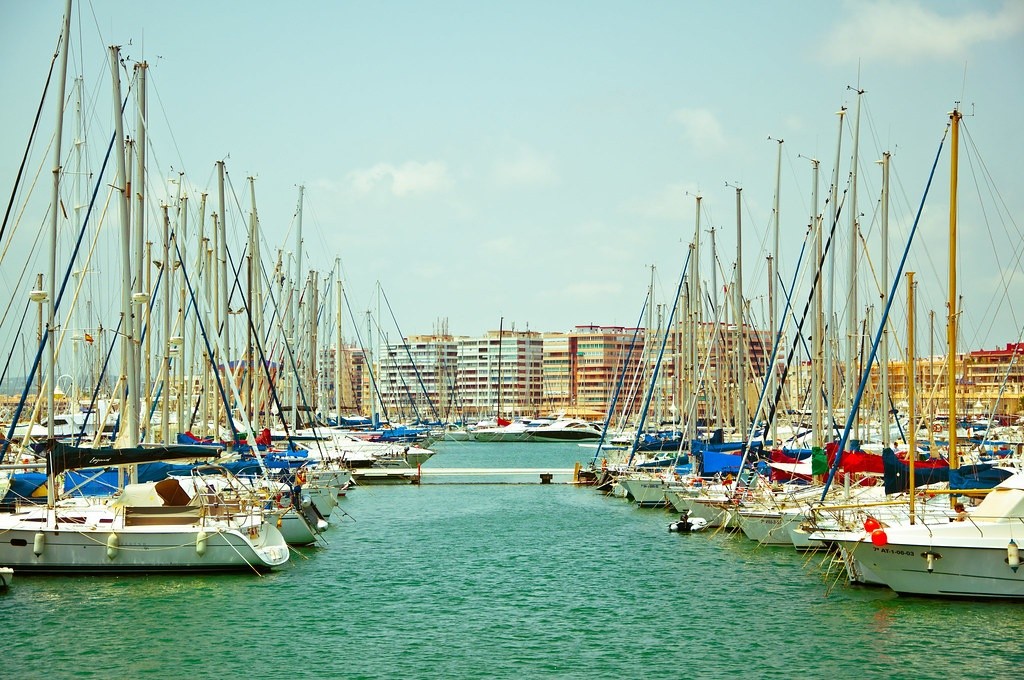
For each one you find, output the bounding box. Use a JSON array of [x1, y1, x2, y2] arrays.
[[580, 74, 1024, 600], [470, 418, 544, 443], [529, 416, 609, 443], [1, 0, 435, 573], [443, 429, 474, 441]]
